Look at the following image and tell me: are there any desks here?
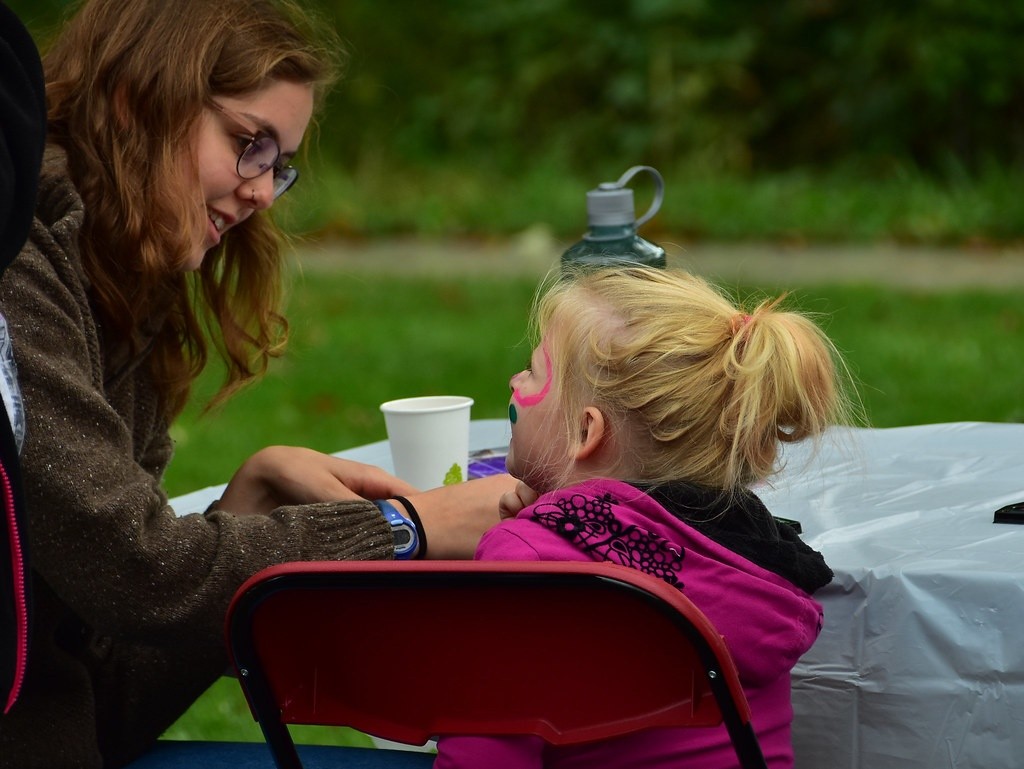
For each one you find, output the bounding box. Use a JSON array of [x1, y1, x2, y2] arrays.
[[162, 418, 1024, 769]]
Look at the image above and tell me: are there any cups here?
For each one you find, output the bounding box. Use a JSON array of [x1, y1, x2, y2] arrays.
[[378, 395, 475, 493]]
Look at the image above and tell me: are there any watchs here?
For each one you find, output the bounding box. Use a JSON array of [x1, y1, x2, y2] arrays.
[[372, 499, 418, 560]]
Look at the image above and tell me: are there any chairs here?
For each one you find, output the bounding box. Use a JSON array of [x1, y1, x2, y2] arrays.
[[221, 559, 766, 769]]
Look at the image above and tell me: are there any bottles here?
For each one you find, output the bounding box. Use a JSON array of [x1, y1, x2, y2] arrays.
[[561, 165, 667, 280]]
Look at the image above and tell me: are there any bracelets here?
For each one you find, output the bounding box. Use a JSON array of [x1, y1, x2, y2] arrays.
[[390, 495, 428, 560]]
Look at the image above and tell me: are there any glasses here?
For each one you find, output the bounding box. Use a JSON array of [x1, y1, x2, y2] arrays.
[[205, 96, 299, 202]]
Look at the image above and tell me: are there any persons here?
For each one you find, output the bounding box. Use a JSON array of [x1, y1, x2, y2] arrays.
[[432, 253, 881, 769], [0, 0, 523, 769]]
